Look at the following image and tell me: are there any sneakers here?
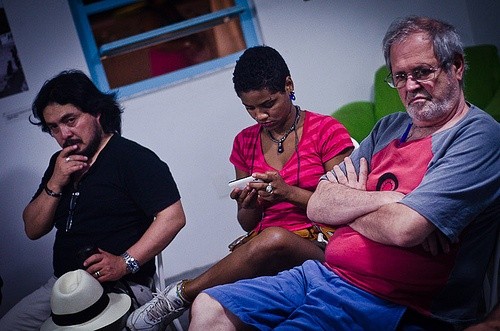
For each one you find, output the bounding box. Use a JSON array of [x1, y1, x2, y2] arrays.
[[126, 279, 190, 331]]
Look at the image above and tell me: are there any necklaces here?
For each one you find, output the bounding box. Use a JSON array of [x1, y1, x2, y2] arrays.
[[65, 165, 90, 233], [265, 105, 300, 153]]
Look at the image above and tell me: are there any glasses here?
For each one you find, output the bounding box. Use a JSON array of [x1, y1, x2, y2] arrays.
[[65, 191, 79, 232], [384, 61, 445, 89]]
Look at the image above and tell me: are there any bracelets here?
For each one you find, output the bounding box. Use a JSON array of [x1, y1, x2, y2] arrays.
[[45, 184, 63, 197]]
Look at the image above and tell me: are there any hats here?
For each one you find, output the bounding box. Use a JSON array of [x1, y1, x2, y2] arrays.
[[39, 270, 131, 331]]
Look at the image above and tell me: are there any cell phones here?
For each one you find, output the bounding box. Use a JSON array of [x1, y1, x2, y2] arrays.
[[227, 174, 264, 193]]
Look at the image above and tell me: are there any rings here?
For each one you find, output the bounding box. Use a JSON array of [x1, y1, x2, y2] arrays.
[[265, 183, 273, 194], [95, 271, 101, 278], [65, 156, 70, 162]]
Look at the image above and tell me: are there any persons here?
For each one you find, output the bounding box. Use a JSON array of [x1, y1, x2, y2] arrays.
[[126, 45, 354, 331], [0, 68, 186, 331], [188, 13, 500, 331]]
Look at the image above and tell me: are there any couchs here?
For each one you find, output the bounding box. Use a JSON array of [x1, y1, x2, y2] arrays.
[[328, 44, 500, 146]]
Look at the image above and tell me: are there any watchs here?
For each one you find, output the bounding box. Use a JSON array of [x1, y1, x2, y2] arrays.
[[121, 252, 140, 274]]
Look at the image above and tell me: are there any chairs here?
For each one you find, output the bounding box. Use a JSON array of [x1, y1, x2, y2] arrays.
[[153, 251, 184, 331]]
